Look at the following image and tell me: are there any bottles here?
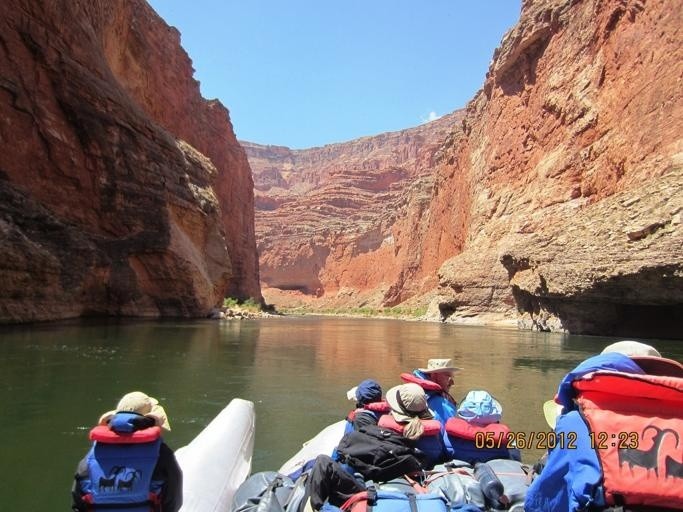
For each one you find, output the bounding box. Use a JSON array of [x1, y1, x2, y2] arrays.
[[470, 457, 505, 500]]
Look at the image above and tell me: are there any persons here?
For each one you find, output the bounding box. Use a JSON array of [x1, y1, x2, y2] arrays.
[[376, 381, 441, 467], [72, 391, 184, 512], [345, 380, 385, 435], [410, 357, 459, 424], [525, 340, 682, 511], [441, 390, 518, 463]]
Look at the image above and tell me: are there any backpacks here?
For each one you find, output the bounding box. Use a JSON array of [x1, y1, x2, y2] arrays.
[[337, 424, 417, 481]]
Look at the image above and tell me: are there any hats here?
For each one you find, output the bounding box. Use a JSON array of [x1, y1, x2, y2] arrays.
[[599, 340, 683, 376], [356, 379, 382, 403], [386, 382, 429, 415], [117, 391, 159, 414], [418, 359, 464, 374], [459, 390, 502, 422], [543, 399, 563, 430]]
